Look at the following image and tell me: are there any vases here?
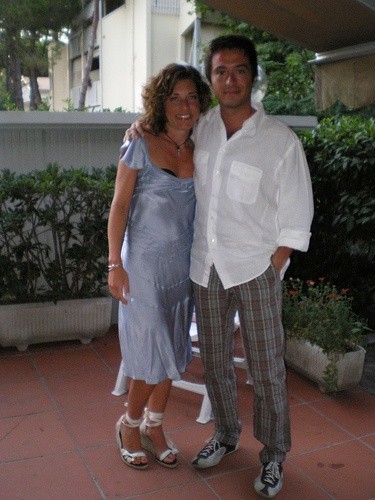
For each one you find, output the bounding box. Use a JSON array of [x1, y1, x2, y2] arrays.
[[284, 332, 365, 392]]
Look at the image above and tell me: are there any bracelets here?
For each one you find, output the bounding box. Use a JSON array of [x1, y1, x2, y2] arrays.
[[108, 264, 123, 271]]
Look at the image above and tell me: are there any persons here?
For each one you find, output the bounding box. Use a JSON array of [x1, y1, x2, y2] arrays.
[[106, 64, 213, 469], [124, 34, 314, 498]]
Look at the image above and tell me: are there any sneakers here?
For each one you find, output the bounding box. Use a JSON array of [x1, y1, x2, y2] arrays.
[[254, 462, 284, 499], [191, 437, 241, 469]]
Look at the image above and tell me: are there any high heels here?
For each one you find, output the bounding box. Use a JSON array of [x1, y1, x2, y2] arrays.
[[115, 402, 150, 469], [139, 407, 180, 469]]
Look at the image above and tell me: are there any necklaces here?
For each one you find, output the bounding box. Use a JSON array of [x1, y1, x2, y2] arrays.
[[165, 132, 185, 156]]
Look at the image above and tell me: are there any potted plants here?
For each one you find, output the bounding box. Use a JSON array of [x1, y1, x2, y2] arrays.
[[0, 163, 125, 352]]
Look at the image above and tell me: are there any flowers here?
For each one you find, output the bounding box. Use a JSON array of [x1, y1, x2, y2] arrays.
[[279, 274, 370, 396]]
